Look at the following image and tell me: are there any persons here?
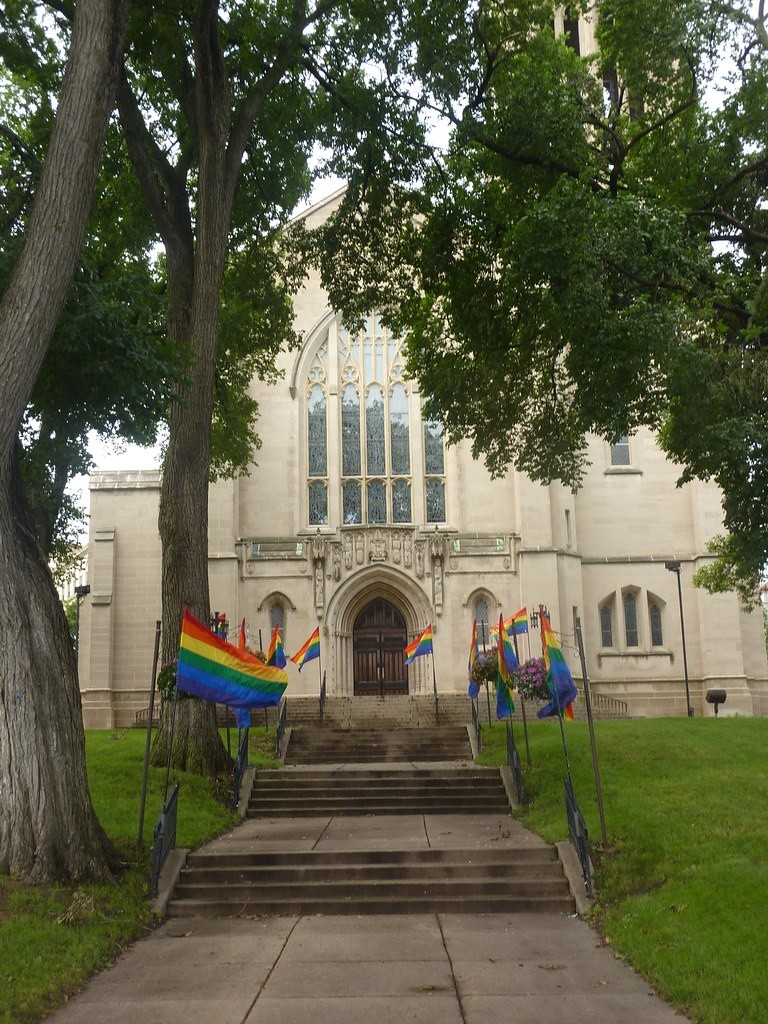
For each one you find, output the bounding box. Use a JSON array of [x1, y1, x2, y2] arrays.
[[332, 548, 341, 581], [404, 534, 412, 568], [393, 534, 402, 564], [344, 536, 352, 569], [355, 536, 364, 565], [415, 546, 424, 578]]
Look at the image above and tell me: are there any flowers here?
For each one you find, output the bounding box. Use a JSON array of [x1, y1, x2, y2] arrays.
[[468, 646, 498, 685], [504, 655, 548, 700], [157, 656, 188, 701], [247, 647, 269, 664]]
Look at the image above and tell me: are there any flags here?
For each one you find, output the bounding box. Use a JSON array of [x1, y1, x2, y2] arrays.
[[216, 611, 227, 638], [496, 611, 519, 720], [534, 603, 578, 721], [263, 624, 287, 669], [289, 626, 321, 675], [232, 617, 252, 730], [176, 604, 289, 709], [466, 618, 480, 698], [488, 607, 529, 646], [402, 623, 432, 665]]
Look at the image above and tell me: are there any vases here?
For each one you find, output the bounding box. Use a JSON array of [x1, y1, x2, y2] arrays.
[[536, 685, 552, 700], [485, 669, 498, 682]]
